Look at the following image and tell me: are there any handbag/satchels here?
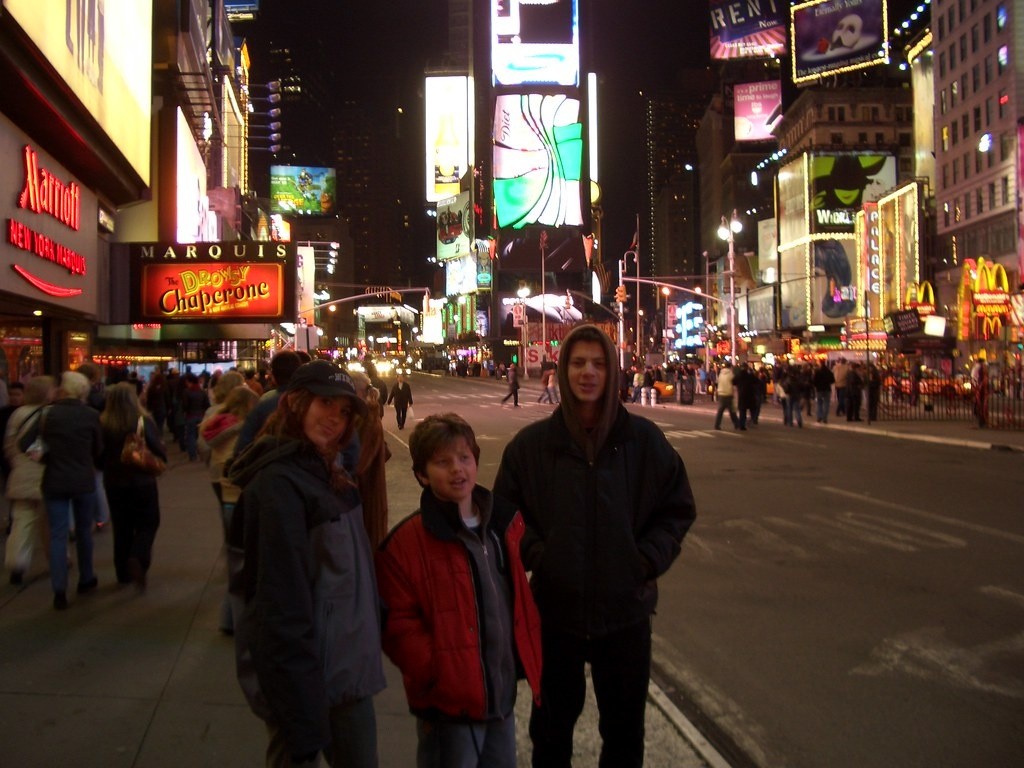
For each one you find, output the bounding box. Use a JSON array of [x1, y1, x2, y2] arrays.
[[25, 406, 46, 463], [120, 414, 167, 477]]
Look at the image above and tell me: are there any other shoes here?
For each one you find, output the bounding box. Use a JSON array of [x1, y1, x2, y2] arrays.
[[9, 571, 24, 588], [96, 521, 107, 531], [53, 596, 75, 610], [78, 575, 97, 593]]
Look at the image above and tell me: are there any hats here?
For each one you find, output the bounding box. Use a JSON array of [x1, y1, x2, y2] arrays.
[[291, 360, 368, 418]]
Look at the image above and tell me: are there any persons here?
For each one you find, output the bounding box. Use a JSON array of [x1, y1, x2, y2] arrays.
[[0, 361, 209, 609], [200, 352, 387, 768], [971, 358, 989, 427], [387, 374, 413, 430], [381, 413, 542, 768], [907, 361, 923, 407], [448, 357, 506, 380], [697, 356, 881, 430], [492, 325, 699, 768]]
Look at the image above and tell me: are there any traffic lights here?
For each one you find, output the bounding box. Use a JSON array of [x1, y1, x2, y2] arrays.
[[615, 285, 627, 303], [512, 354, 517, 364]]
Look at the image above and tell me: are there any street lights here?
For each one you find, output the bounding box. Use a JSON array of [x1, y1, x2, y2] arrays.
[[662, 287, 669, 362], [718, 209, 742, 366], [618, 251, 637, 369], [517, 288, 529, 380], [703, 251, 710, 375]]
